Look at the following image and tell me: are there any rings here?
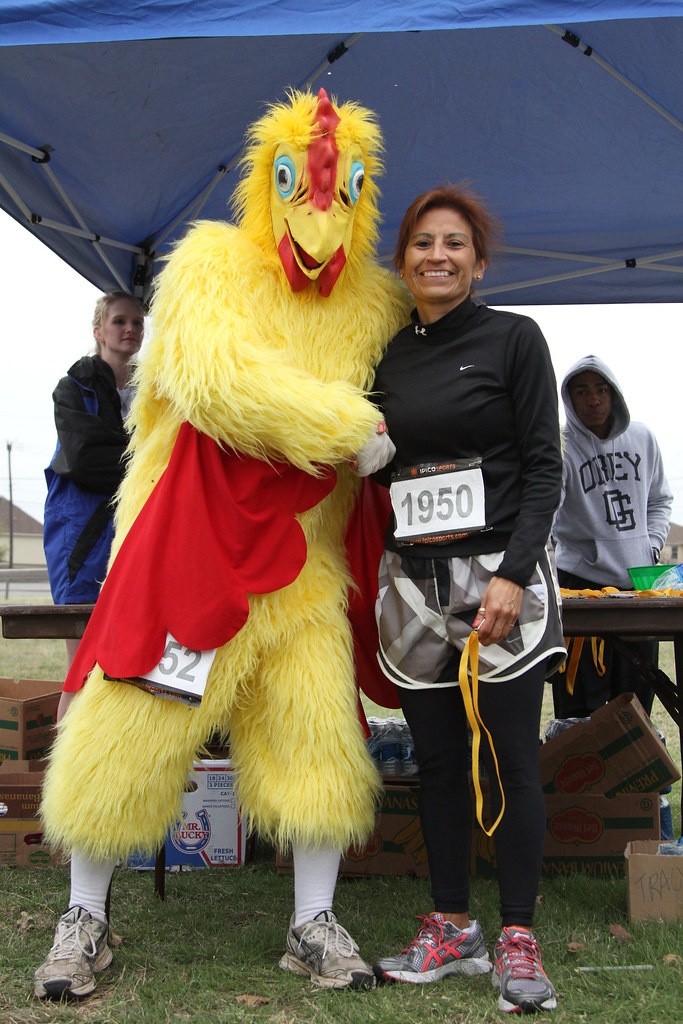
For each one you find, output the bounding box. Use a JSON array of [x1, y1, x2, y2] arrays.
[[511, 623, 515, 628], [478, 607, 486, 613]]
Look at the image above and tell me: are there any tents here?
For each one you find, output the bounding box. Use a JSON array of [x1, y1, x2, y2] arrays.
[[0, 0, 683, 306]]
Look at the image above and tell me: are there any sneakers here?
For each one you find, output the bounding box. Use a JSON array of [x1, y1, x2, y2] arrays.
[[492, 927, 555, 1011], [281, 911, 375, 990], [32, 906, 114, 994], [375, 912, 497, 982]]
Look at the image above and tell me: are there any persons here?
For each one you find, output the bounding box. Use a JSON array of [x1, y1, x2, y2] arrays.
[[32, 86, 416, 999], [42, 292, 134, 737], [546, 353, 672, 717], [363, 178, 570, 1015]]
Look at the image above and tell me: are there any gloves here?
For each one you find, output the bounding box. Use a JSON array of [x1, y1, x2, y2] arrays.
[[353, 430, 396, 479]]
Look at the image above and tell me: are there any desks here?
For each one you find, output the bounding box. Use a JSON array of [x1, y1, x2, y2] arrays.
[[2, 597, 683, 905]]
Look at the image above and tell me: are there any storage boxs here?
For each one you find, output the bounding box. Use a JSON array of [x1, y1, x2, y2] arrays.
[[1, 678, 679, 873], [623, 838, 683, 924]]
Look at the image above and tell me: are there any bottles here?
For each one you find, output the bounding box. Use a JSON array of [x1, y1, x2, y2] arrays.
[[365, 716, 420, 777]]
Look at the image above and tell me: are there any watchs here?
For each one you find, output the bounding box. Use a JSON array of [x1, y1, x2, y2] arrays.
[[653, 549, 660, 564]]
